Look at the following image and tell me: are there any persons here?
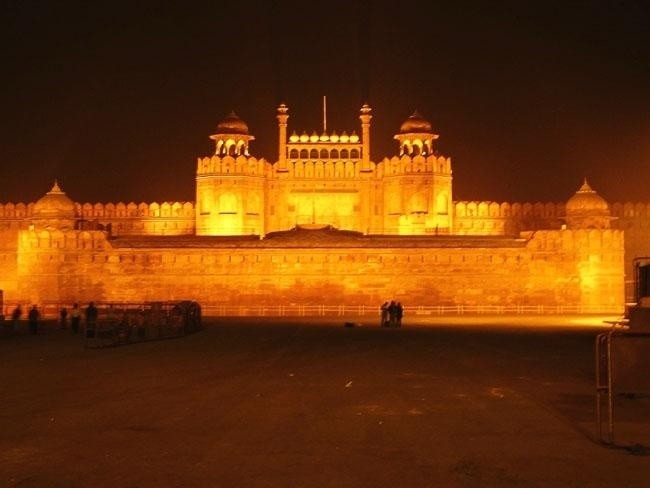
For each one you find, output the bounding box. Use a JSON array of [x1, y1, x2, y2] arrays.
[[84, 302, 98, 330], [379, 300, 404, 328], [28, 305, 40, 336], [69, 302, 80, 335], [60, 307, 67, 330], [8, 304, 22, 335]]
